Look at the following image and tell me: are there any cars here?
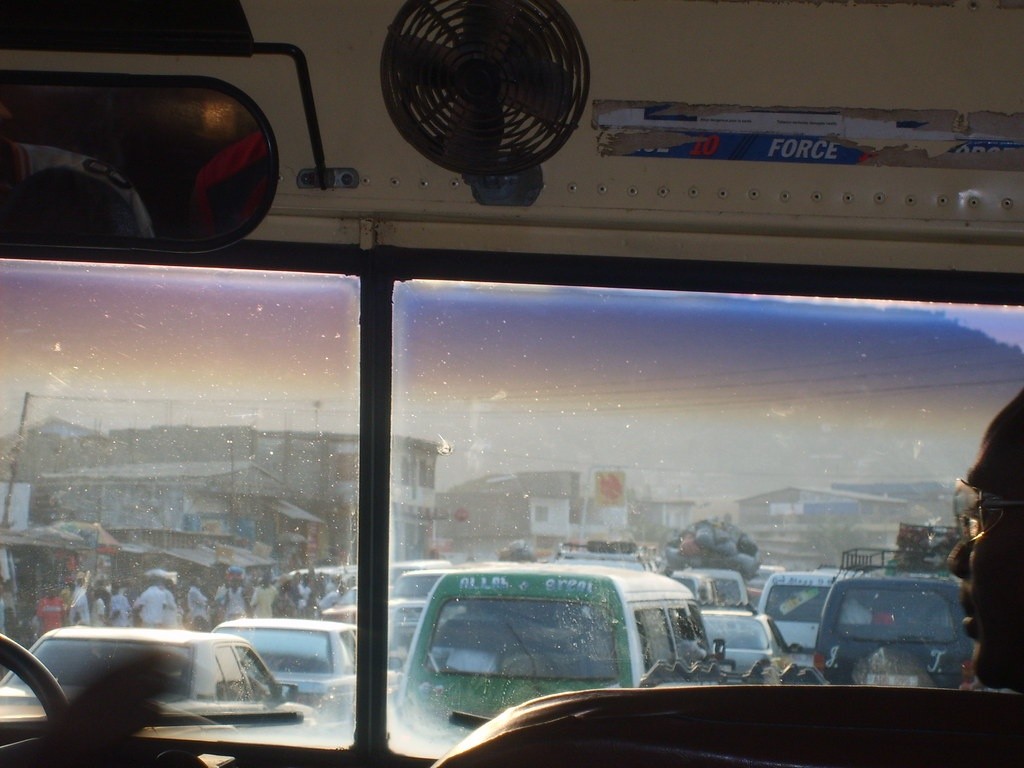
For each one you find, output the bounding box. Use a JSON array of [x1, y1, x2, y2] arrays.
[[275, 558, 808, 687], [0, 624, 320, 756], [207, 614, 395, 729]]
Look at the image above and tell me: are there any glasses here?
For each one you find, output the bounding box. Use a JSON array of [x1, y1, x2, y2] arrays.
[[953, 478, 1024, 546]]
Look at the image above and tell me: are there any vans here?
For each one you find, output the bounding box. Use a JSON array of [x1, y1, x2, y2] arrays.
[[755, 569, 846, 655], [809, 570, 976, 696], [388, 556, 737, 735]]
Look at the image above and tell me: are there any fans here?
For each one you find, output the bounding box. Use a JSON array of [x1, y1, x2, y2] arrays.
[[380, 0, 590, 207]]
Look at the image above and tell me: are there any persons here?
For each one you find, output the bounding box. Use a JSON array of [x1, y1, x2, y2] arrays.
[[0, 126, 270, 242], [0, 564, 351, 768], [947, 385, 1024, 699]]
